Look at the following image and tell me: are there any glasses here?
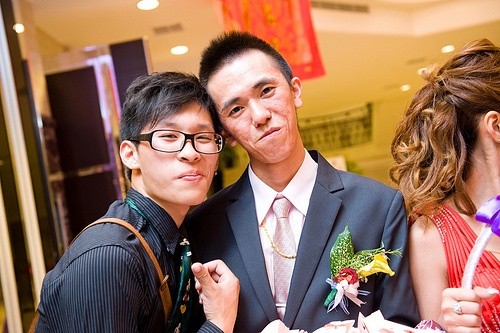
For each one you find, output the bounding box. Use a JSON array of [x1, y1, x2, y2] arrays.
[[128, 128, 224, 155]]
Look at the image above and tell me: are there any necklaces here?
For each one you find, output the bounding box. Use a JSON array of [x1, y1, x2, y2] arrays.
[[261, 221, 297, 259]]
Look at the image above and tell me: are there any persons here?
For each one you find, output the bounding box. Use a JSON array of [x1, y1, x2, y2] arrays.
[[35, 72, 240, 333], [183, 30, 421, 333], [389, 38, 500, 333]]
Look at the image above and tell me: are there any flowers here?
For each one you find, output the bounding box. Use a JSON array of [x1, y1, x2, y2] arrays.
[[323, 223, 403, 315]]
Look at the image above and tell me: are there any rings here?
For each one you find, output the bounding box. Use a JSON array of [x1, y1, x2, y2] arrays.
[[453, 301, 463, 315]]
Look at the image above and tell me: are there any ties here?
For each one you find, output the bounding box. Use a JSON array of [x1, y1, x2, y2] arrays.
[[125, 194, 193, 333], [271, 196, 297, 321]]
